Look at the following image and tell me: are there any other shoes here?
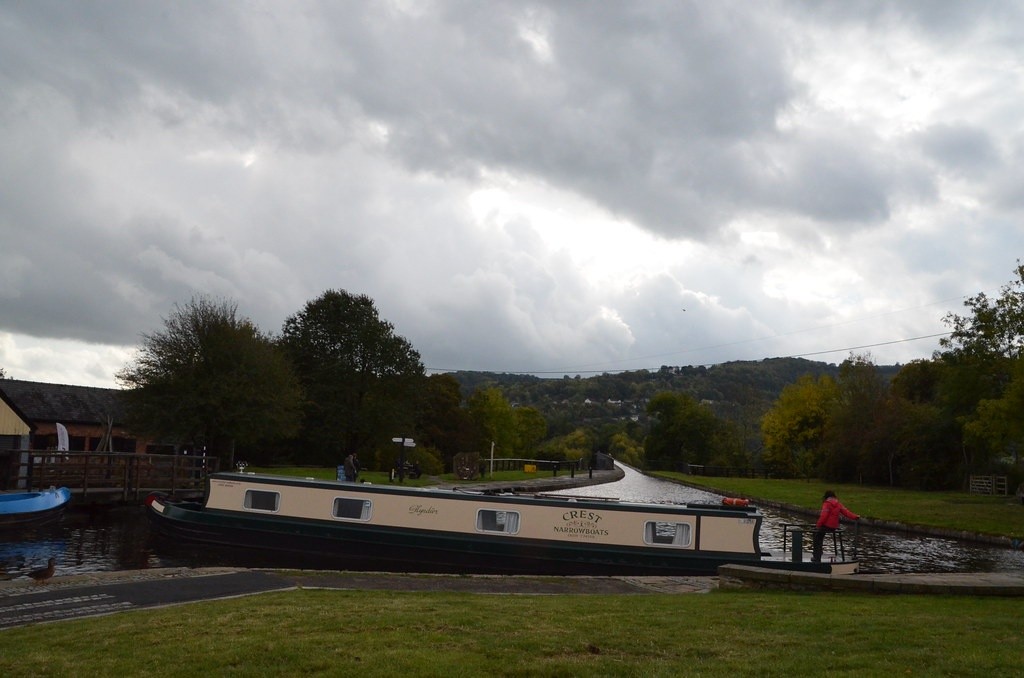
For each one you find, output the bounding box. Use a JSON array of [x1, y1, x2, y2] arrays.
[[811, 556, 821, 561]]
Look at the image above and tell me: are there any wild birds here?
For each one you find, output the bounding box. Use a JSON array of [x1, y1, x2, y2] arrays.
[[22, 557, 57, 585]]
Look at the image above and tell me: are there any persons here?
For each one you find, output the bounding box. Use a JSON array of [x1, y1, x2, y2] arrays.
[[811, 490, 860, 562], [344, 452, 361, 482], [415, 460, 422, 478]]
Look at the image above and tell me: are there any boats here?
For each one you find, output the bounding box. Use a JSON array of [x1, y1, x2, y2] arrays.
[[146, 471, 859, 575], [0, 487, 71, 525]]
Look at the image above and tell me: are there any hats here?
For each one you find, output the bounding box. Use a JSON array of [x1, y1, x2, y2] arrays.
[[823, 491, 837, 499]]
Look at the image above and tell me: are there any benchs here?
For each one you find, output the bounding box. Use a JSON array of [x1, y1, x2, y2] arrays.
[[779, 523, 846, 563]]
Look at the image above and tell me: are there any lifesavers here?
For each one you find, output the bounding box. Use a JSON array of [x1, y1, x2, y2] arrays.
[[725, 498, 750, 506]]
